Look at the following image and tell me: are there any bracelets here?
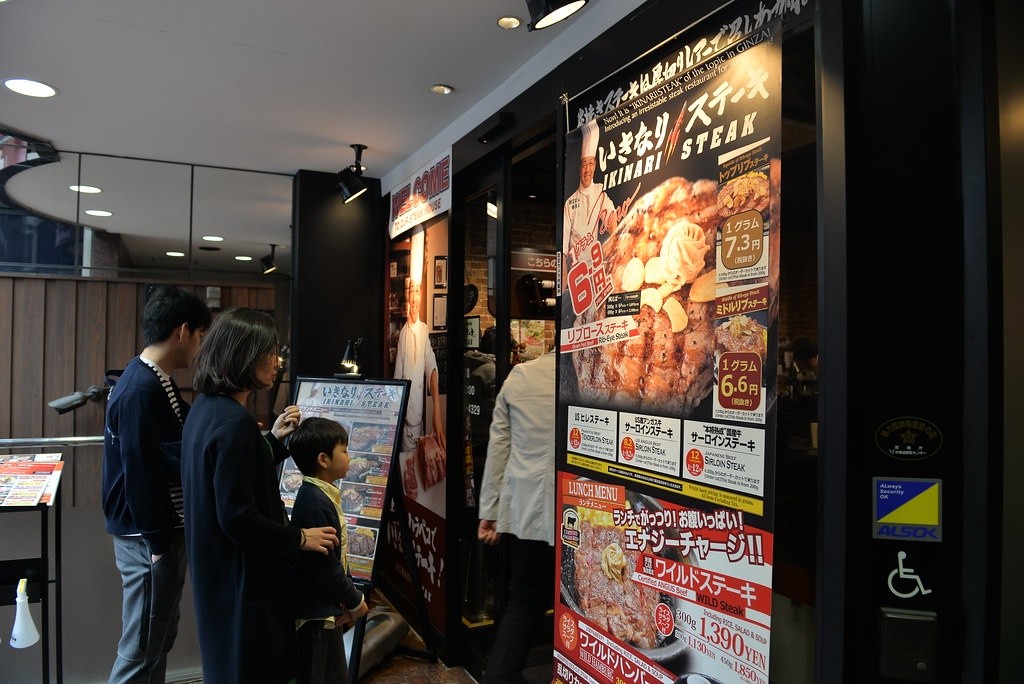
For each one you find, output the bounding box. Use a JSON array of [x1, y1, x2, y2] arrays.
[[300, 529, 307, 548]]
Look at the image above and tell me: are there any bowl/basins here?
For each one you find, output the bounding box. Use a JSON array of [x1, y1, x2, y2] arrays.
[[560, 477, 700, 662], [283, 474, 303, 494], [351, 427, 384, 452]]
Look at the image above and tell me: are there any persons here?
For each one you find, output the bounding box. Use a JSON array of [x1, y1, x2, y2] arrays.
[[561, 119, 617, 290], [180, 306, 367, 684], [393, 225, 447, 453], [101, 286, 213, 684], [478, 346, 556, 684]]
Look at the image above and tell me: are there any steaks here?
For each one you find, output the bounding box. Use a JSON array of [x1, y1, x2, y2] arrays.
[[571, 175, 717, 420], [573, 520, 662, 651]]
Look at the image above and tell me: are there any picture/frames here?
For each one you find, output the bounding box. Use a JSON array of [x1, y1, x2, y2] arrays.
[[434, 256, 447, 289], [432, 294, 446, 331]]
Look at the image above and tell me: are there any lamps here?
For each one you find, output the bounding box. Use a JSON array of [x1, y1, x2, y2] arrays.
[[260, 245, 277, 274], [48, 386, 104, 416], [333, 144, 369, 204], [524, 0, 589, 31]]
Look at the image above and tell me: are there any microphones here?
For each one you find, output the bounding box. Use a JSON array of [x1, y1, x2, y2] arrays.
[[87, 386, 102, 402]]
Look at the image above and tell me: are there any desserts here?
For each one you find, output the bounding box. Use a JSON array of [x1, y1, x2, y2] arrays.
[[600, 543, 626, 582], [659, 219, 708, 285]]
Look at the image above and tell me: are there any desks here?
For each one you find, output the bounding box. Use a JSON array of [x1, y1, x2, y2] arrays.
[[0, 460, 64, 684]]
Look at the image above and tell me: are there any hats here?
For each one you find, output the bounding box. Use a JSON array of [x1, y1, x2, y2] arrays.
[[410, 223, 424, 282], [581, 119, 599, 160]]
